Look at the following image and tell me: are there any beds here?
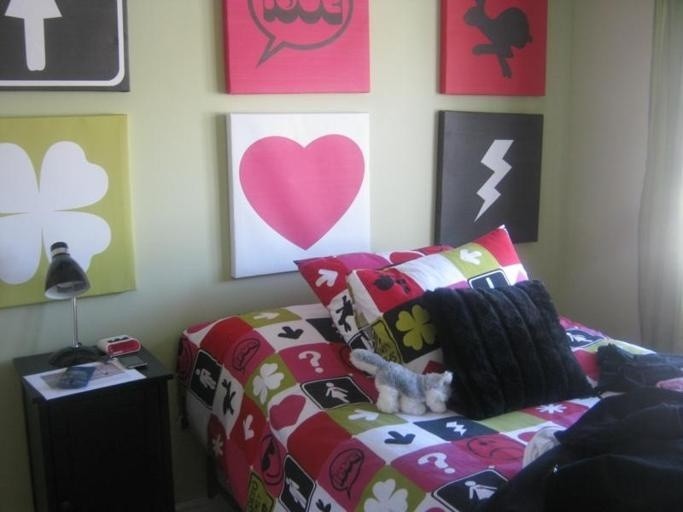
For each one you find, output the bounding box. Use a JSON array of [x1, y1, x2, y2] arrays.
[[178, 300, 681, 510]]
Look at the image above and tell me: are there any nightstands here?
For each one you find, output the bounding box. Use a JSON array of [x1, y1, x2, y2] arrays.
[[11, 338, 182, 511]]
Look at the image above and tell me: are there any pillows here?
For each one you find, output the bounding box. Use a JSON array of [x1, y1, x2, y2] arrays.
[[422, 279, 601, 421], [345, 224, 529, 375], [292, 243, 457, 379]]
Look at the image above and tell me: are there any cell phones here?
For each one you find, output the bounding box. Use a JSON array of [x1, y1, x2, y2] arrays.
[[118, 355, 147, 369]]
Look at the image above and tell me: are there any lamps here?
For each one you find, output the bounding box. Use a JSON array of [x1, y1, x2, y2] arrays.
[[42, 240, 99, 369]]
